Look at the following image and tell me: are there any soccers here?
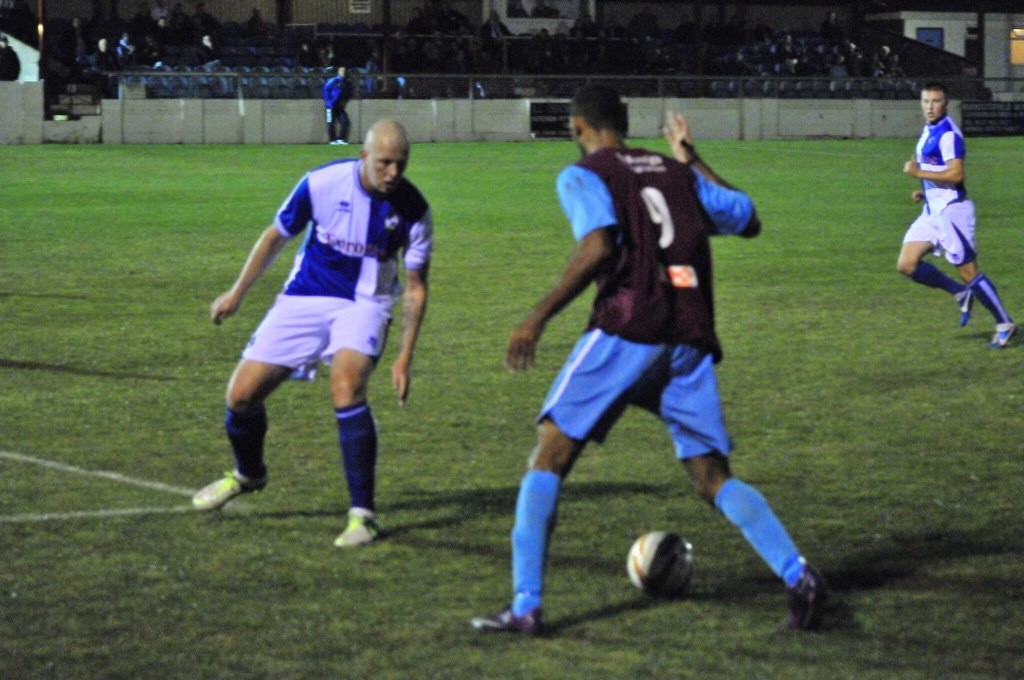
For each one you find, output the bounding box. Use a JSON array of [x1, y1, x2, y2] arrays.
[[625, 530, 696, 598]]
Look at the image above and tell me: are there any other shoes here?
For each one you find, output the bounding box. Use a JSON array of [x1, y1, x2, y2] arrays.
[[337, 139, 348, 145], [330, 141, 339, 145]]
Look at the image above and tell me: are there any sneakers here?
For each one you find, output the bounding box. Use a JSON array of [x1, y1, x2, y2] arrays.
[[786, 564, 828, 632], [988, 321, 1017, 349], [468, 605, 542, 635], [332, 506, 378, 547], [954, 288, 973, 327], [192, 464, 269, 511]]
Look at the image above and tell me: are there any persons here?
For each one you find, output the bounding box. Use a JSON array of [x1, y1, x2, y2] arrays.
[[819, 12, 842, 37], [248, 7, 270, 38], [469, 85, 831, 631], [777, 34, 798, 61], [726, 51, 752, 76], [0, 0, 222, 99], [191, 117, 433, 546], [482, 10, 512, 52], [297, 42, 317, 68], [360, 0, 899, 98], [319, 42, 337, 67], [896, 82, 1018, 349], [323, 67, 353, 145]]
